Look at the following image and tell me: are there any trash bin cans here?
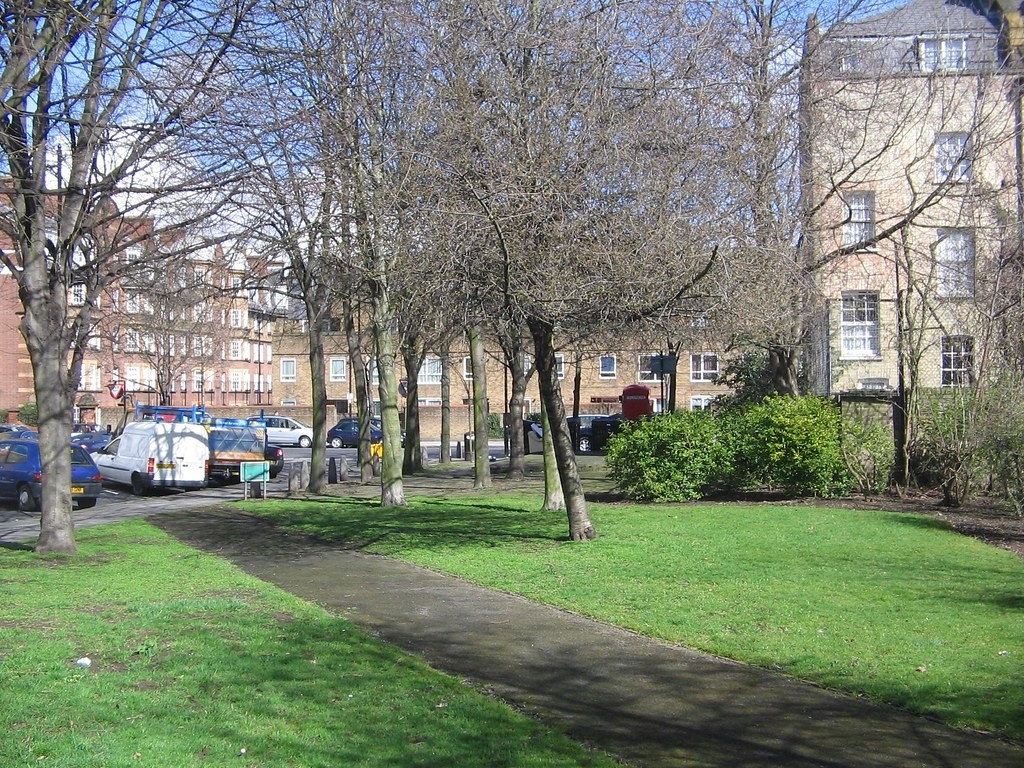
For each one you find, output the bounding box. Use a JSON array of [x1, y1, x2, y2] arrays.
[[591, 416, 623, 454], [371, 443, 383, 474], [464, 431, 476, 461]]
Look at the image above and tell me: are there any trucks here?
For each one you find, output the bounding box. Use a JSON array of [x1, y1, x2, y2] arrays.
[[89, 420, 211, 495], [135, 399, 267, 485]]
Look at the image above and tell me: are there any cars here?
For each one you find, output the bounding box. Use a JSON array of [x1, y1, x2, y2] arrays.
[[566, 414, 609, 454], [264, 444, 286, 479], [0, 420, 118, 455], [1, 441, 101, 512], [247, 416, 313, 448], [327, 417, 406, 448]]
